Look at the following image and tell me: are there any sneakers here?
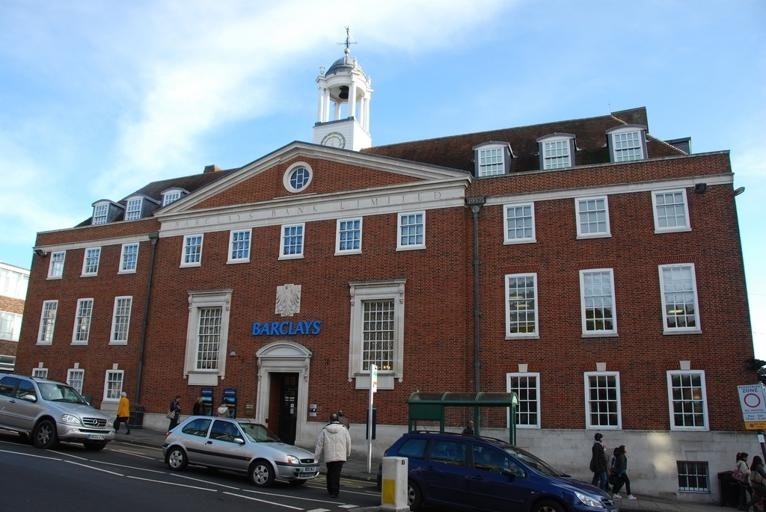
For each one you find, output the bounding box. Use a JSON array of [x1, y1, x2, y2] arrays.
[[613, 495, 623, 499], [628, 495, 637, 500]]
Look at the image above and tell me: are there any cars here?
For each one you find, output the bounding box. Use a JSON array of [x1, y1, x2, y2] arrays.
[[0, 372, 116, 453], [160, 415, 320, 488], [377, 430, 620, 512]]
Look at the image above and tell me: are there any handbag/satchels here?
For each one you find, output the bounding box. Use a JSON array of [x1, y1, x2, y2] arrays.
[[113, 419, 120, 430], [732, 468, 744, 482], [167, 409, 176, 420], [749, 470, 766, 486]]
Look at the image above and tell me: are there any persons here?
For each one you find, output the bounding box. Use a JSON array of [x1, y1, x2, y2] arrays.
[[111, 390, 131, 435], [313, 413, 352, 498], [742, 455, 766, 512], [590, 432, 608, 490], [167, 395, 184, 429], [192, 397, 206, 415], [335, 409, 350, 428], [461, 419, 474, 434], [612, 445, 638, 499], [217, 399, 229, 416], [610, 447, 621, 495], [736, 450, 754, 510]]
